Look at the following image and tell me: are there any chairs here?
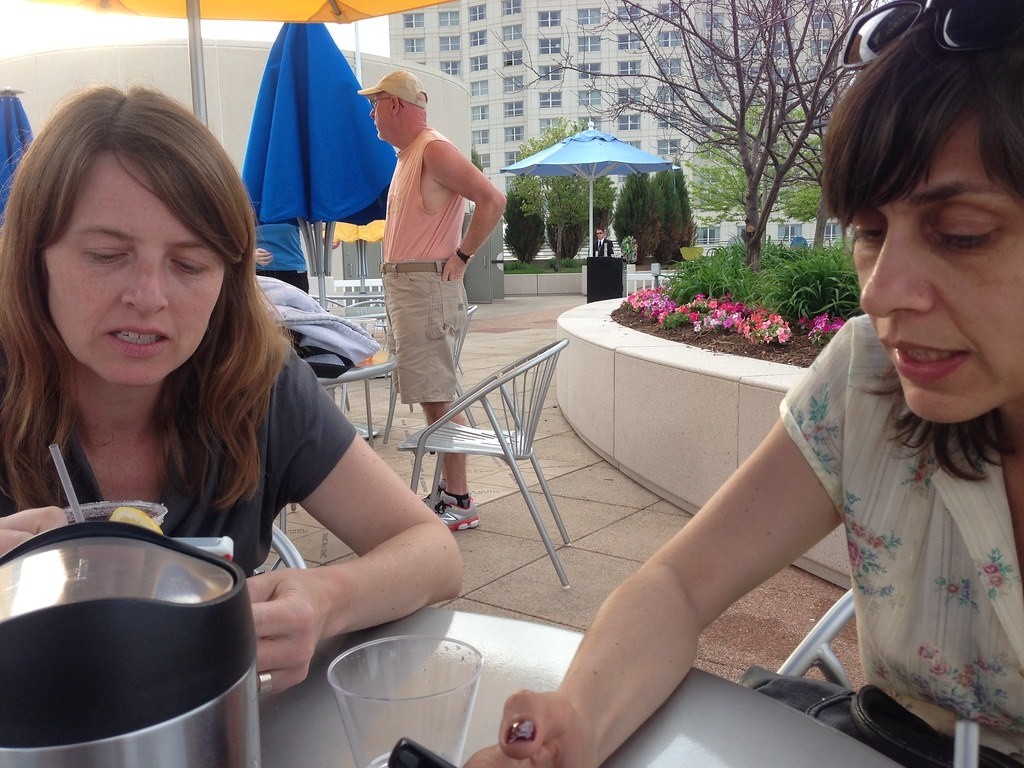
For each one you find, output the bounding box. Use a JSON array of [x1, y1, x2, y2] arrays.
[[452, 304, 478, 374], [397, 336, 572, 590]]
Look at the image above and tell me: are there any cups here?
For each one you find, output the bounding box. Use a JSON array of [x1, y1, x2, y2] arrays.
[[63, 500, 168, 528], [327, 634, 484, 768]]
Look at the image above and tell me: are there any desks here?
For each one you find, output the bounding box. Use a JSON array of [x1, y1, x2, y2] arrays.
[[218, 609, 903, 768], [316, 348, 395, 451], [327, 307, 391, 342], [311, 290, 386, 308]]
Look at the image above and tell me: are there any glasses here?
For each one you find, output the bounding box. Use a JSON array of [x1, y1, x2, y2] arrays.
[[370, 98, 404, 109], [596, 232, 603, 235], [841, 1, 1022, 69]]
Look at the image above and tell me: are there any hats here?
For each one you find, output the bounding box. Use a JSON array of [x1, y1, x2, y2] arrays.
[[356, 70, 429, 109]]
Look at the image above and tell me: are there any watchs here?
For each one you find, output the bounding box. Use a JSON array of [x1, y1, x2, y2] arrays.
[[455, 247, 475, 264]]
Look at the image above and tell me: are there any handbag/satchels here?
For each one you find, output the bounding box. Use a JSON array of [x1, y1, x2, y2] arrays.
[[803, 682, 1024, 768]]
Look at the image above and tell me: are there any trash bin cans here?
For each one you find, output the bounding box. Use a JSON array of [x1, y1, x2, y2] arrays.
[[586, 257, 628, 303]]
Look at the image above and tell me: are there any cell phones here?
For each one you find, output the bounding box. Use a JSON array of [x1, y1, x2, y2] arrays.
[[172, 536, 234, 563], [389, 738, 457, 768]]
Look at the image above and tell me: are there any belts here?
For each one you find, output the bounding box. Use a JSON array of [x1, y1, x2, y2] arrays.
[[379, 263, 444, 273]]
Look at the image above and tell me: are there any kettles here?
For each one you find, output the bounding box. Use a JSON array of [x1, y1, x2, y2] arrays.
[[0, 521, 261, 767]]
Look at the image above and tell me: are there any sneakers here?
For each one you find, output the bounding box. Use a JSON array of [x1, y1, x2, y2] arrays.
[[421, 478, 447, 508], [432, 490, 481, 531]]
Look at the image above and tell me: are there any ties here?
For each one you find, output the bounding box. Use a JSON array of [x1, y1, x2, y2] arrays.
[[598, 240, 601, 249]]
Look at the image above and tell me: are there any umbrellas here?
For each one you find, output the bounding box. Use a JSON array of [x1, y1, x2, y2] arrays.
[[243, 22, 396, 313], [334, 221, 386, 293], [25, 0, 455, 126], [499, 121, 672, 257], [0, 88, 33, 222]]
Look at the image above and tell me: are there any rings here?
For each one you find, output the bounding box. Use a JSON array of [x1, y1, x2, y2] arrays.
[[259, 673, 272, 693]]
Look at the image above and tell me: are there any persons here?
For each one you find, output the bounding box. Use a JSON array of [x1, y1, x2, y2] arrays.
[[458, 0, 1023, 768], [593, 228, 614, 257], [252, 222, 339, 293], [355, 72, 508, 532], [0, 85, 464, 699]]
